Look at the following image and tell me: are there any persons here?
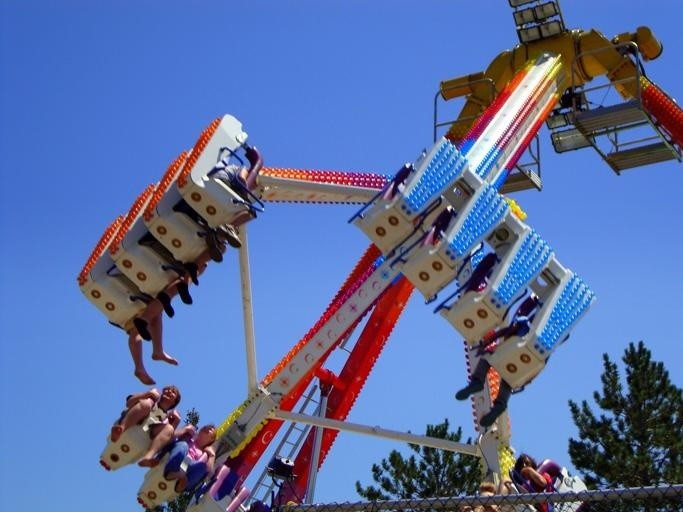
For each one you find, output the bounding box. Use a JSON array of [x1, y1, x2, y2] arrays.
[[122, 163, 262, 384], [158, 418, 218, 495], [452, 289, 568, 427], [459, 481, 498, 512], [109, 380, 183, 469], [480, 452, 553, 511]]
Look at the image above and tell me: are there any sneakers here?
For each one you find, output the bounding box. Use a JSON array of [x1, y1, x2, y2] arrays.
[[218, 223, 242, 248], [479, 401, 504, 427], [455, 381, 482, 400], [205, 235, 223, 263]]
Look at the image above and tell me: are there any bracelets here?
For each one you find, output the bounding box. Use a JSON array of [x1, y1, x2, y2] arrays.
[[208, 453, 215, 457]]
